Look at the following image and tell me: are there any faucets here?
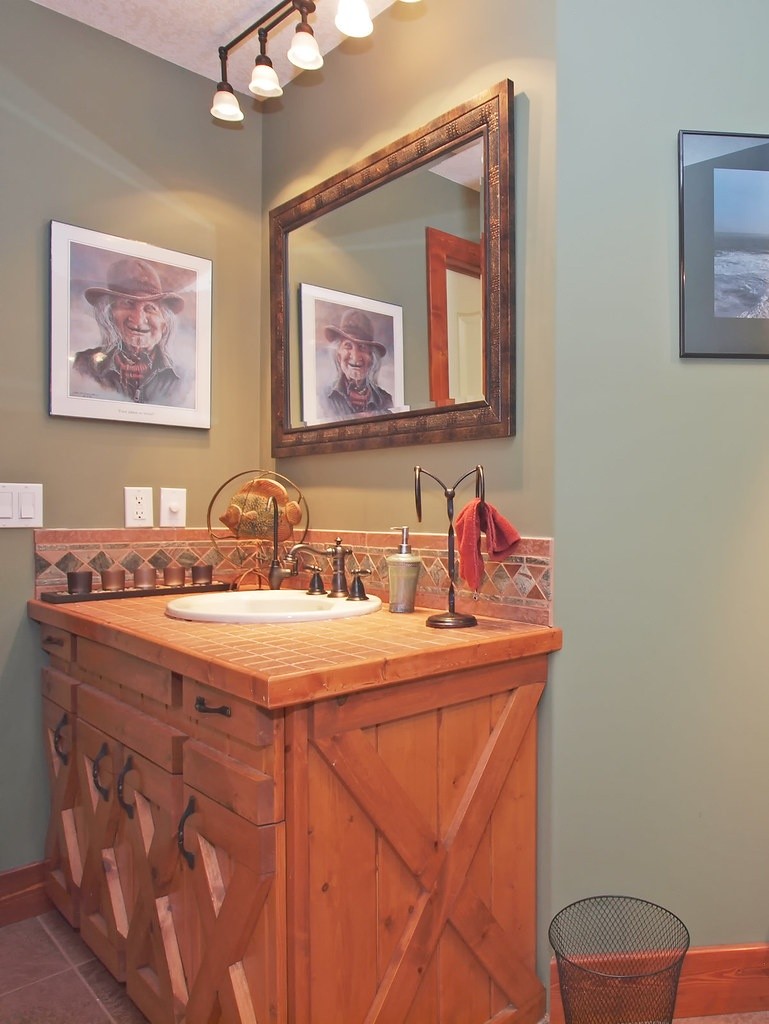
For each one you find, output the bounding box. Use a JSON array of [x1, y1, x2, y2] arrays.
[[285, 536, 353, 598], [265, 495, 298, 590]]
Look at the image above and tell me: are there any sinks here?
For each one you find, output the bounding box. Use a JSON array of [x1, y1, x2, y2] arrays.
[[165, 589, 381, 623]]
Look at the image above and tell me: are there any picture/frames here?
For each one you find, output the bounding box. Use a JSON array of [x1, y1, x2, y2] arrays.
[[677, 130, 769, 359], [48, 216, 214, 432], [300, 282, 405, 422]]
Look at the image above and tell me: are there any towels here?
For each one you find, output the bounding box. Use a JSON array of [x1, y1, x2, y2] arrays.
[[453, 497, 521, 602]]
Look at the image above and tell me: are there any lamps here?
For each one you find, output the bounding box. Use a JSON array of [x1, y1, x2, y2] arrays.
[[210, 0, 424, 121]]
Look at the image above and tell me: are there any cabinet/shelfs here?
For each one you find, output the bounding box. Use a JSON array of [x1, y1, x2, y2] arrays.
[[39, 622, 81, 930], [182, 654, 547, 1024], [76, 637, 183, 1024]]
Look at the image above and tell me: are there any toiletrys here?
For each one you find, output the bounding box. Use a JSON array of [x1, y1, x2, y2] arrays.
[[384, 525, 422, 613]]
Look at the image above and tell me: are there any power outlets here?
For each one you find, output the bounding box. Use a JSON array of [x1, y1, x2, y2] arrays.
[[124, 487, 153, 528]]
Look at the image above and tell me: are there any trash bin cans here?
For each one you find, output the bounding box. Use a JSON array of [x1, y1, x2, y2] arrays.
[[548, 895, 691, 1024]]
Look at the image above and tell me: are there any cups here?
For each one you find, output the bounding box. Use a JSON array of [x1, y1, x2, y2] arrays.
[[101, 570, 125, 590], [133, 568, 156, 588], [192, 565, 213, 584], [164, 567, 186, 586], [67, 572, 92, 593]]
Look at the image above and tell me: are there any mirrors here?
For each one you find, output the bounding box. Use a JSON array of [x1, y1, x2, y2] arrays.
[[270, 78, 516, 457]]
